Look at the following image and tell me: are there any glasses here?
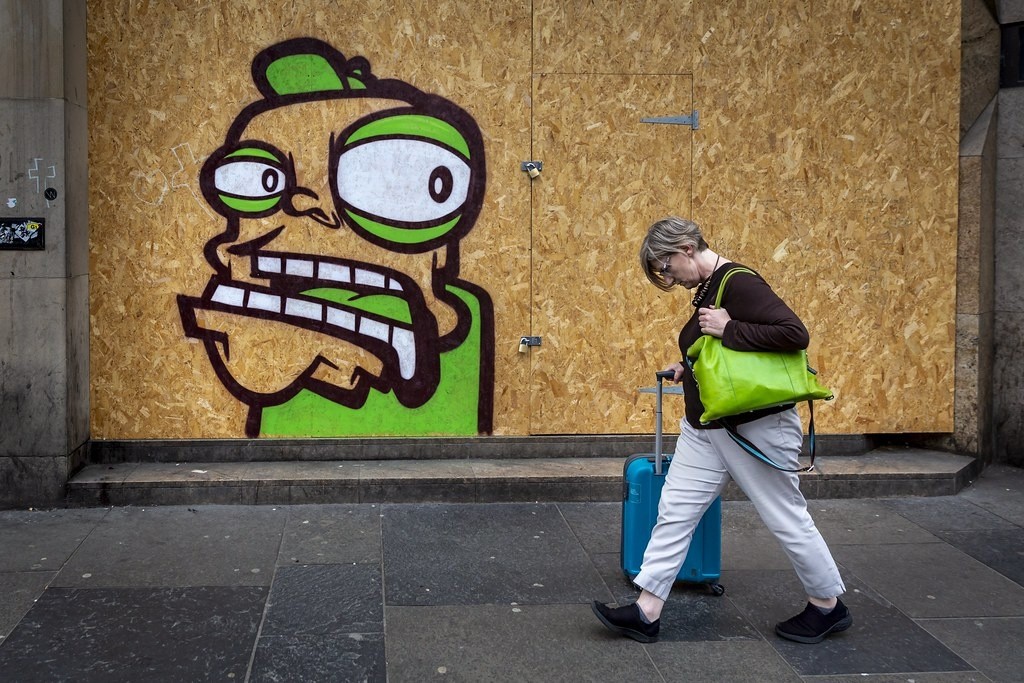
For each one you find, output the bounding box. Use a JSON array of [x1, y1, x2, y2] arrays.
[[659, 253, 672, 276]]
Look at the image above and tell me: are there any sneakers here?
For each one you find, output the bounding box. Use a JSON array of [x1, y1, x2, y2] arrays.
[[591, 600, 660, 643], [775, 597, 852, 643]]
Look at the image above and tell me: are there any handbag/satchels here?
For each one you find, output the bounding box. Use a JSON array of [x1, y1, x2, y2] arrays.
[[687, 268, 833, 422]]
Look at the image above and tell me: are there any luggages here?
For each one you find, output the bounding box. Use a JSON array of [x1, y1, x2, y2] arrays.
[[620, 370, 725, 596]]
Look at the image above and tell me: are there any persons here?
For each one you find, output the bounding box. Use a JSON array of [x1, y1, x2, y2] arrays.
[[590, 216, 852, 643]]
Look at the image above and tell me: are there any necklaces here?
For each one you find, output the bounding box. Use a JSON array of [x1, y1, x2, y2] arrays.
[[692, 254, 721, 307]]
